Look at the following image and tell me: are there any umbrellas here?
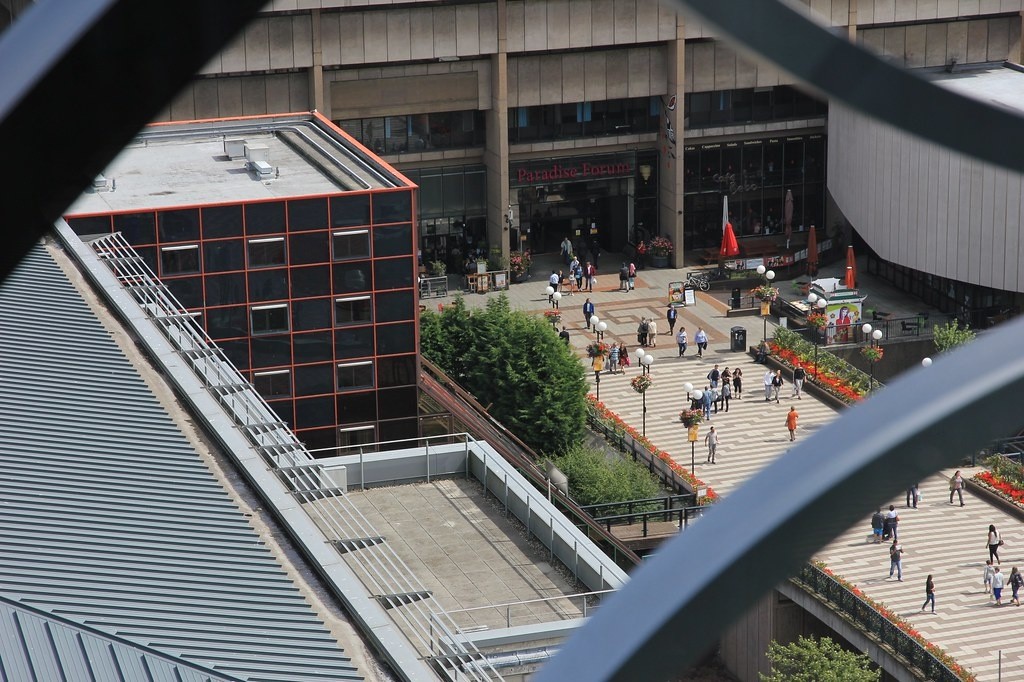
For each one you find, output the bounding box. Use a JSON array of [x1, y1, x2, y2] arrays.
[[719, 221, 740, 256], [844, 244, 857, 289], [806, 225, 819, 286], [784, 189, 793, 249]]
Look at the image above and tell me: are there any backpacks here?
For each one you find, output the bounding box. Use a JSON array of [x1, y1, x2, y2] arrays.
[[1014, 572, 1023, 587], [642, 321, 648, 331]]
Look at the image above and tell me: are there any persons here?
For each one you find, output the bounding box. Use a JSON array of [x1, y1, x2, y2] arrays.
[[638, 240, 647, 269], [609, 342, 629, 375], [675, 327, 688, 358], [636, 316, 657, 348], [559, 326, 569, 346], [619, 261, 629, 293], [583, 298, 594, 329], [701, 362, 807, 465], [667, 304, 677, 336], [550, 268, 564, 292], [451, 247, 460, 256], [590, 239, 600, 266], [584, 261, 596, 294], [578, 239, 587, 260], [466, 258, 477, 270], [986, 525, 1001, 565], [561, 236, 572, 267], [694, 326, 708, 359], [629, 263, 637, 290], [1007, 566, 1024, 607], [871, 470, 965, 583], [569, 256, 583, 297], [983, 560, 1004, 606]]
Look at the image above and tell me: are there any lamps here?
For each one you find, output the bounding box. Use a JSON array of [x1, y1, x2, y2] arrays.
[[639, 165, 650, 180]]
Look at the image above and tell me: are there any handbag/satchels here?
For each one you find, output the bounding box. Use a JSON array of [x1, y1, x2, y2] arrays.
[[609, 354, 611, 359], [895, 510, 899, 521], [560, 249, 563, 255], [999, 540, 1004, 545], [792, 383, 796, 395], [593, 278, 597, 283], [626, 357, 631, 366], [916, 493, 922, 502], [949, 487, 953, 491], [605, 359, 610, 370], [620, 274, 627, 280]]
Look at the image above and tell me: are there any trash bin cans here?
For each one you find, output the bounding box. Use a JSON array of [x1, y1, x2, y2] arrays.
[[731, 327, 746, 352], [732, 289, 740, 309]]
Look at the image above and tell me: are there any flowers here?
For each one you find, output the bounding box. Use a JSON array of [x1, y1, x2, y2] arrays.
[[860, 347, 883, 364], [509, 250, 531, 276], [807, 314, 829, 334], [543, 310, 562, 323], [680, 411, 701, 420], [646, 236, 673, 257], [750, 287, 778, 303], [631, 374, 657, 394], [585, 342, 609, 356]]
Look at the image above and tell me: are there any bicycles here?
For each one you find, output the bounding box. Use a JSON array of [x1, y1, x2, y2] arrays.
[[683, 276, 711, 291]]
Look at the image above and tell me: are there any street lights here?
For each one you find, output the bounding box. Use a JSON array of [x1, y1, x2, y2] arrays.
[[683, 382, 703, 474], [757, 265, 775, 341], [635, 348, 654, 436], [590, 315, 607, 401], [546, 286, 562, 331], [808, 293, 826, 380], [862, 323, 882, 389]]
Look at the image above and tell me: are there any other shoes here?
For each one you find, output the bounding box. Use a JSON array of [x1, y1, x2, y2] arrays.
[[922, 608, 926, 611], [960, 502, 965, 506], [898, 578, 902, 582], [949, 501, 952, 504], [985, 589, 1021, 606], [932, 610, 936, 613]]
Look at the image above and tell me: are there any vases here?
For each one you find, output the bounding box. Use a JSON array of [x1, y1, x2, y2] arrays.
[[638, 387, 648, 393], [549, 317, 558, 322], [685, 420, 696, 428], [866, 356, 879, 364], [651, 254, 671, 268], [809, 327, 820, 341], [759, 293, 771, 301], [509, 268, 529, 284]]
